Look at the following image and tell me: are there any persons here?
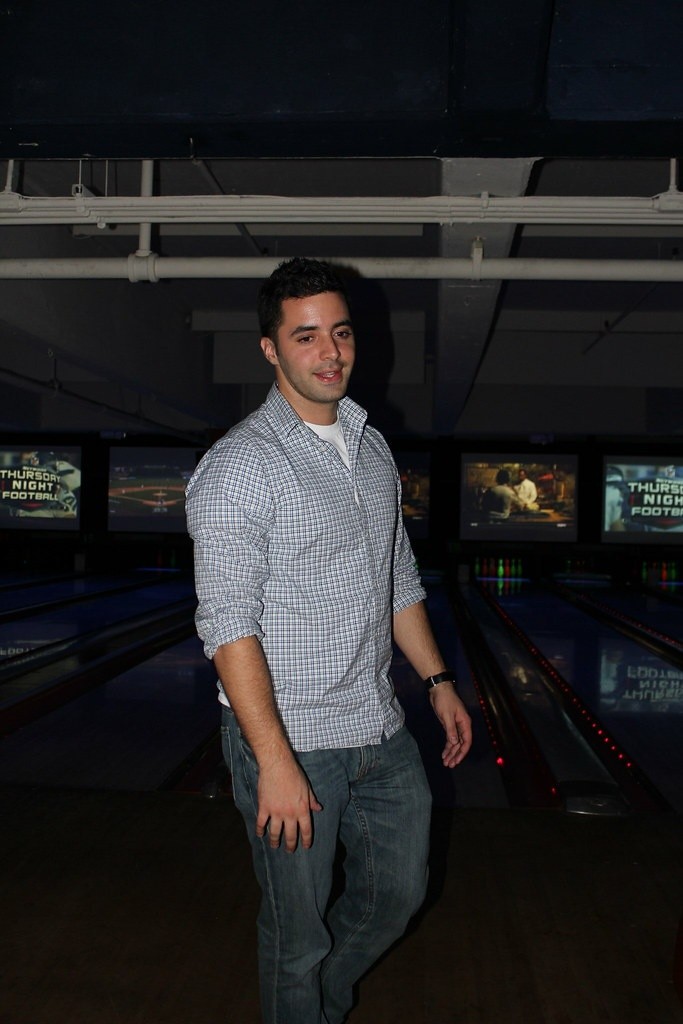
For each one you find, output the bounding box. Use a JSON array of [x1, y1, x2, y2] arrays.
[[483, 468, 539, 522], [183, 256, 472, 1024]]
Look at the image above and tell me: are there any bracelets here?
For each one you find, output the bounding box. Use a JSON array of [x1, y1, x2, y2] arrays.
[[423, 668, 457, 690]]
